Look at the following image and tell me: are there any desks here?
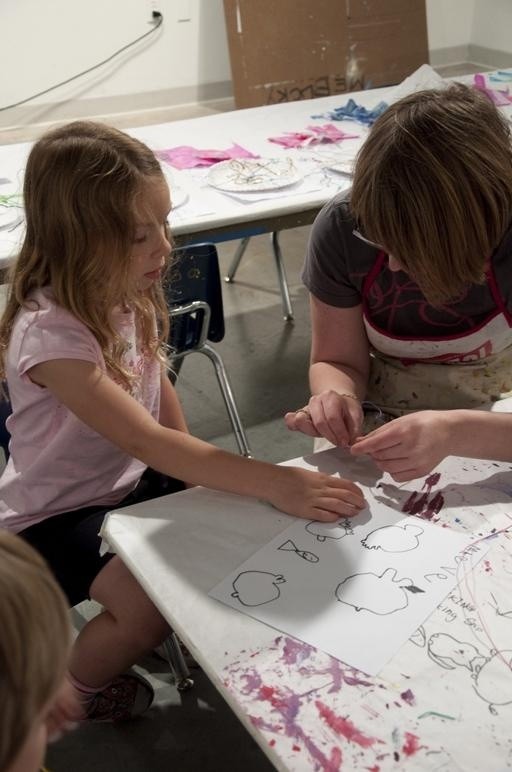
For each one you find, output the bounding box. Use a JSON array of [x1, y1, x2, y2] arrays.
[[99, 394, 512, 772], [0, 67, 512, 321]]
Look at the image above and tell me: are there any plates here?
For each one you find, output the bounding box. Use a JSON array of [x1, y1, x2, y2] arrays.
[[206, 158, 304, 192]]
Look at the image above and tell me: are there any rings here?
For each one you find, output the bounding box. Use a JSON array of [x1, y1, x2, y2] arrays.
[[295, 409, 309, 419]]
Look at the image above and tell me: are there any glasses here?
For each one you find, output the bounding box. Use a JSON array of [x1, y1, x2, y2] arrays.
[[351, 226, 384, 251]]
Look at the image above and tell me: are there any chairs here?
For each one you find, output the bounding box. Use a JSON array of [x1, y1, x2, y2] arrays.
[[161, 242, 251, 462], [0, 383, 195, 693]]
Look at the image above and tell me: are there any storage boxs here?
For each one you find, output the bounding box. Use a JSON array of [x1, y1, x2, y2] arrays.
[[224, 0, 430, 108]]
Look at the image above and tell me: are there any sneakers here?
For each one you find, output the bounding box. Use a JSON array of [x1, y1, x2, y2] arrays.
[[81, 673, 154, 722]]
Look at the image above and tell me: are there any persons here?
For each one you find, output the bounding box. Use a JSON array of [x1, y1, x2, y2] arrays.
[[284, 82, 512, 485], [1, 118, 366, 726], [0, 527, 72, 772]]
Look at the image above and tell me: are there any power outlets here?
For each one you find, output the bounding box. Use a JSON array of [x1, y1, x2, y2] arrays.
[[147, 0, 162, 23]]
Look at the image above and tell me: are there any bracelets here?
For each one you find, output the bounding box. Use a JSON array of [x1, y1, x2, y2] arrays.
[[340, 393, 359, 402]]
